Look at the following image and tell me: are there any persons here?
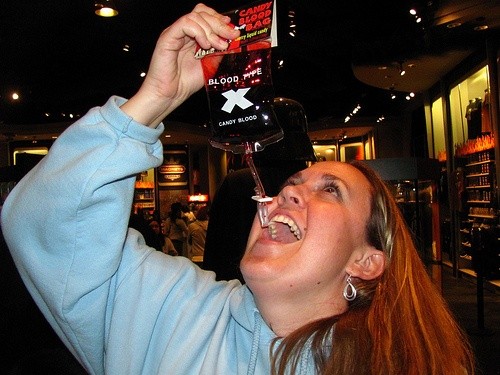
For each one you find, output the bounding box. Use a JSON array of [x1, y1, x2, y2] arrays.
[[1, 3, 471, 375], [203, 98, 315, 280], [130, 202, 206, 266]]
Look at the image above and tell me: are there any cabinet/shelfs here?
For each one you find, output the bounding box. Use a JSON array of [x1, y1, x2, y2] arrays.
[[460, 147, 500, 262]]
